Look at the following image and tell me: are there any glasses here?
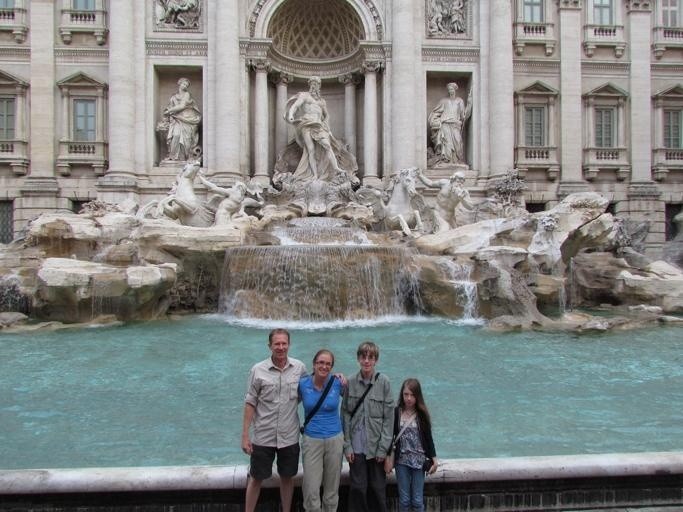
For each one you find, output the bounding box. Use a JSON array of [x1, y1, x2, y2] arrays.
[[314, 361, 332, 366]]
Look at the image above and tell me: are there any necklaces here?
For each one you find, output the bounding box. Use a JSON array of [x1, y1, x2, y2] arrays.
[[404, 409, 416, 417]]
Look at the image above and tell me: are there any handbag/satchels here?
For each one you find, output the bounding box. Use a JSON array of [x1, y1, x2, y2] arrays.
[[384, 447, 396, 473]]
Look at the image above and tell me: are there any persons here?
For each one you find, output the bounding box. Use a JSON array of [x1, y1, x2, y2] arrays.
[[420, 169, 496, 229], [240, 326, 348, 512], [282, 77, 347, 179], [194, 172, 259, 228], [160, 75, 203, 160], [387, 375, 440, 512], [340, 341, 396, 512], [296, 348, 349, 512], [426, 80, 473, 165]]
